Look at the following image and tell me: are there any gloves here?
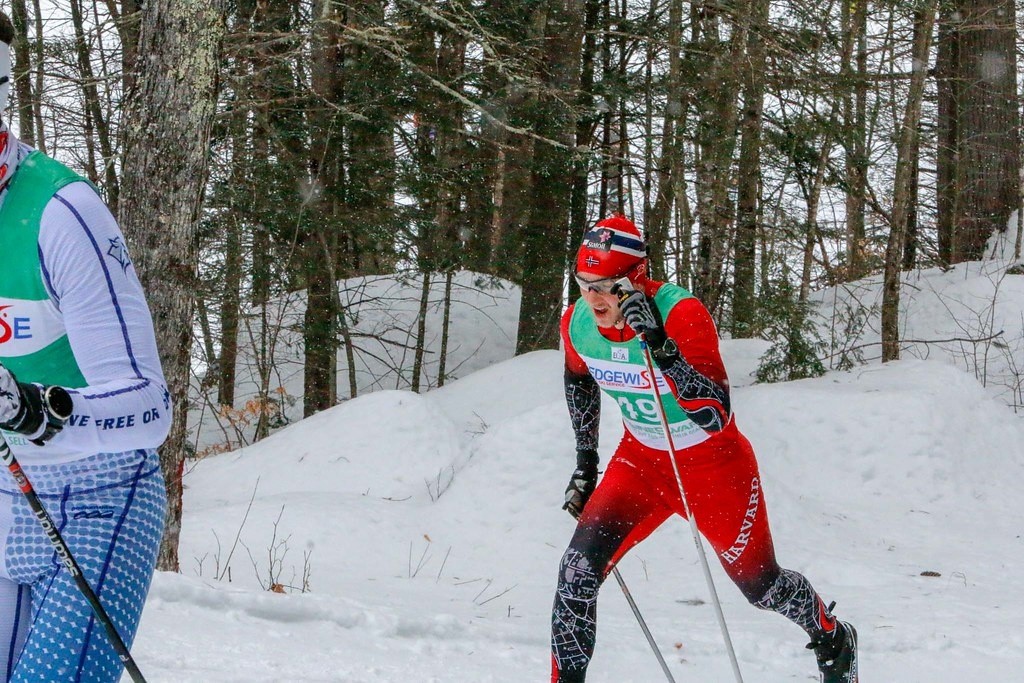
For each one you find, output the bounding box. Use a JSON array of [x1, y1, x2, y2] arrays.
[[564, 463, 603, 518], [617, 289, 668, 351], [0, 363, 44, 437]]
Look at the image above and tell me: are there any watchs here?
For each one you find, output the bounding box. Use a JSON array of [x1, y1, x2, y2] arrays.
[[29, 383, 74, 447], [652, 337, 678, 360]]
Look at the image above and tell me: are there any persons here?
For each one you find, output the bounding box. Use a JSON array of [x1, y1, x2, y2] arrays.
[[0, 10, 174, 683], [550, 213, 858, 683]]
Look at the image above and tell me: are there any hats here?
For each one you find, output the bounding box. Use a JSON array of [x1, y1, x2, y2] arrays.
[[576, 210, 648, 284]]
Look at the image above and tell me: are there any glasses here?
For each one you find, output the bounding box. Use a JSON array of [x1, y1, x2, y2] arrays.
[[574, 270, 631, 296]]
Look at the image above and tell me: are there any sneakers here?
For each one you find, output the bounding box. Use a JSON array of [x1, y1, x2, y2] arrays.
[[805, 620, 859, 683]]
